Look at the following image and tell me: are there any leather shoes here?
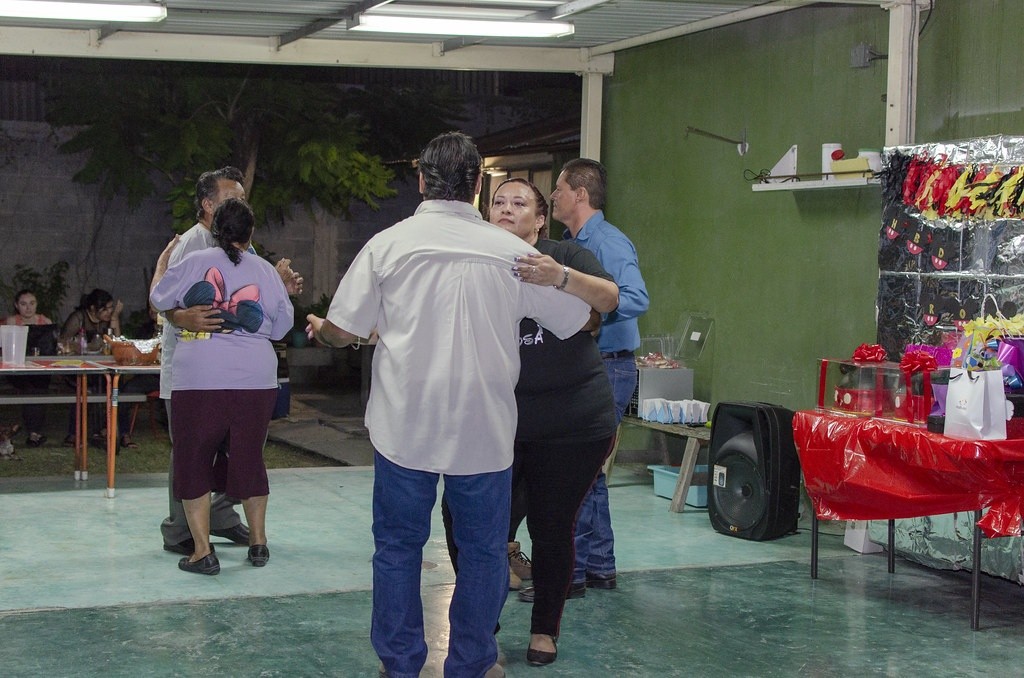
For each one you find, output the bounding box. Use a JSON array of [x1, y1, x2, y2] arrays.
[[584, 570, 617, 589], [164, 536, 215, 557], [521, 580, 587, 601], [210, 522, 250, 544]]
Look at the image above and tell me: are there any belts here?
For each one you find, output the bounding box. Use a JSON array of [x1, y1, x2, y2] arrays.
[[600, 348, 636, 359]]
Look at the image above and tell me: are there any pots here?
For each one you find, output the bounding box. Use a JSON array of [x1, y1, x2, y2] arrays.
[[103, 329, 160, 365]]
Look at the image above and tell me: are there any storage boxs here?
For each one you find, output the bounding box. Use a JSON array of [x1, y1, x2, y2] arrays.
[[644, 459, 708, 509], [813, 357, 900, 423], [874, 360, 950, 433], [842, 520, 885, 554], [926, 412, 945, 433]]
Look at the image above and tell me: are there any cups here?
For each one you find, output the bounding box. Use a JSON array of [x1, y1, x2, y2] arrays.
[[32, 348, 39, 356], [56, 329, 80, 354], [822, 144, 842, 181], [859, 149, 882, 184]]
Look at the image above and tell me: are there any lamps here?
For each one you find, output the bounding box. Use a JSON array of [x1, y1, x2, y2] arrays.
[[340, 11, 577, 43], [0, 0, 171, 25]]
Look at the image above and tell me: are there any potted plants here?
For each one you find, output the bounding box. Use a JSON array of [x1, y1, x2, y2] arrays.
[[288, 292, 333, 348]]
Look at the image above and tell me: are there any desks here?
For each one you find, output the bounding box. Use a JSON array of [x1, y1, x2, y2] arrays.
[[0, 355, 113, 499], [791, 406, 1024, 633], [87, 358, 163, 499]]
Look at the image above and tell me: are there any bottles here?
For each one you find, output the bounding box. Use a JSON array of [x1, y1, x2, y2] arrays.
[[80, 327, 88, 355], [832, 150, 846, 162], [154, 331, 162, 364], [94, 329, 113, 355]]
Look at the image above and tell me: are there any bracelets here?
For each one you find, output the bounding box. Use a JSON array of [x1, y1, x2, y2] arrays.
[[351, 337, 361, 350], [554, 265, 569, 290]]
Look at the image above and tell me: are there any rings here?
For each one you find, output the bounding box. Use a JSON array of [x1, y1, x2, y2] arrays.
[[532, 266, 536, 273]]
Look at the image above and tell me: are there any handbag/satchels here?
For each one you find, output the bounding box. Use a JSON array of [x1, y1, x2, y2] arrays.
[[942, 294, 1024, 440]]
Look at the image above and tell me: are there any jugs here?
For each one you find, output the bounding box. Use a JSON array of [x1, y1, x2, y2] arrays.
[[0, 325, 28, 366]]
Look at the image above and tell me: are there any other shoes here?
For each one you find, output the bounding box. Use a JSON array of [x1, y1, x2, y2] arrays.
[[527, 634, 558, 664], [248, 544, 269, 566], [26, 432, 47, 447], [377, 660, 388, 678], [178, 550, 219, 576], [119, 433, 140, 449], [483, 663, 505, 678], [63, 431, 77, 446]]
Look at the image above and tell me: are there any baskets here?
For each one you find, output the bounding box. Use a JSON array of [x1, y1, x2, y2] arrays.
[[102, 334, 161, 366]]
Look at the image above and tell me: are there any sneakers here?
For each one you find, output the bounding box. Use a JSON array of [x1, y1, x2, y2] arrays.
[[507, 541, 533, 579], [507, 563, 522, 588]]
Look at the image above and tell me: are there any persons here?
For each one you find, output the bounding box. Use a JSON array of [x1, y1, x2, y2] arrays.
[[520, 158, 649, 602], [160, 165, 303, 558], [62, 289, 137, 448], [306, 133, 602, 678], [442, 179, 619, 663], [506, 500, 533, 588], [1, 289, 54, 447], [149, 196, 292, 575]]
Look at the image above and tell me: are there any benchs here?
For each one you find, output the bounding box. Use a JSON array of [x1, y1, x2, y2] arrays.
[[603, 411, 711, 514], [0, 392, 147, 436]]
